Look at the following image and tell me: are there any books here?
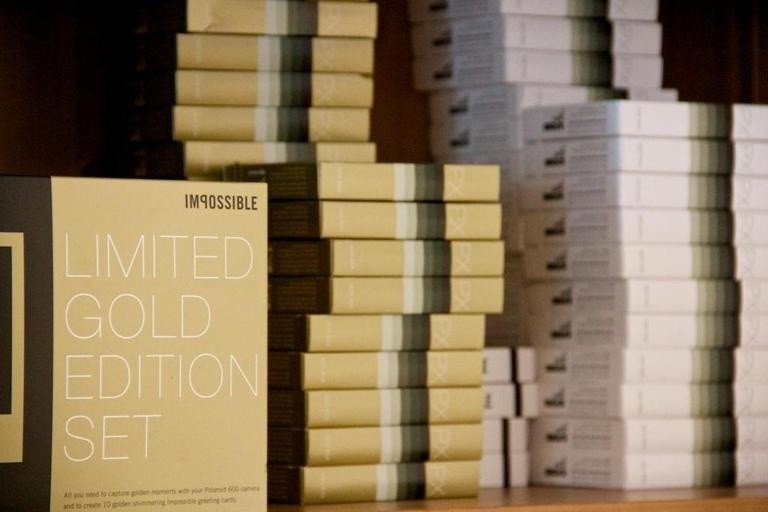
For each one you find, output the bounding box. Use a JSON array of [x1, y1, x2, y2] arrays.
[[2, 0, 766, 511]]
